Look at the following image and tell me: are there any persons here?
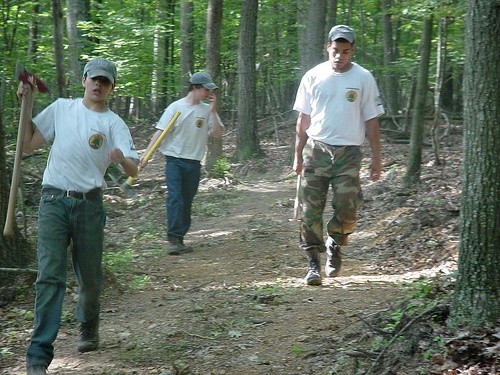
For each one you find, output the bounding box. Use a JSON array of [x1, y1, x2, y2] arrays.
[[136, 72, 223, 255], [293, 24, 381, 286], [16, 56, 138, 375]]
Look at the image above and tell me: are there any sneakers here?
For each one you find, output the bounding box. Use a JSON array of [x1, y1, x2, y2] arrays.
[[78, 314, 99, 352], [27, 365, 46, 375], [168, 237, 193, 255]]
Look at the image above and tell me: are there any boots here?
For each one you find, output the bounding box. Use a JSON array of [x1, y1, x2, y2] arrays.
[[325, 233, 342, 277], [306, 248, 321, 285]]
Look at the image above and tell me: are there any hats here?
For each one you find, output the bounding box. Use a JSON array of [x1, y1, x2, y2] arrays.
[[328, 25, 355, 44], [189, 72, 219, 89], [83, 57, 117, 89]]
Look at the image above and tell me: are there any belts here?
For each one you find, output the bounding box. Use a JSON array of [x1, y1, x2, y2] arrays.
[[42, 189, 100, 199]]
